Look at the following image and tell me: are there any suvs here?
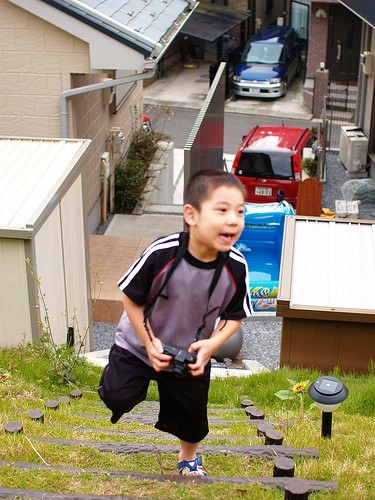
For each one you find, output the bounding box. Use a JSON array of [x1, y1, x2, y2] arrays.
[[232, 25, 302, 100], [229, 124, 324, 215]]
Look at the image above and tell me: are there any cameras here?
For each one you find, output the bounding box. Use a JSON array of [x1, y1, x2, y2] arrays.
[[160, 342, 196, 379]]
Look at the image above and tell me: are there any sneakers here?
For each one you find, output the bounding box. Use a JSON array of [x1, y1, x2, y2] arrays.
[[176, 456, 207, 477]]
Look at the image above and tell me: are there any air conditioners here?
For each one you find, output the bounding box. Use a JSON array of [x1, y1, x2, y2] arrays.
[[338, 125, 369, 173]]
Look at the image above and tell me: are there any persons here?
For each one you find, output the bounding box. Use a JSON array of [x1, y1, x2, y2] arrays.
[[97, 168, 255, 478]]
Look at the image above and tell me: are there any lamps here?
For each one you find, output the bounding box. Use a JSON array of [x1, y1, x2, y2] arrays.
[[320, 61, 325, 71], [308, 376, 349, 439]]
